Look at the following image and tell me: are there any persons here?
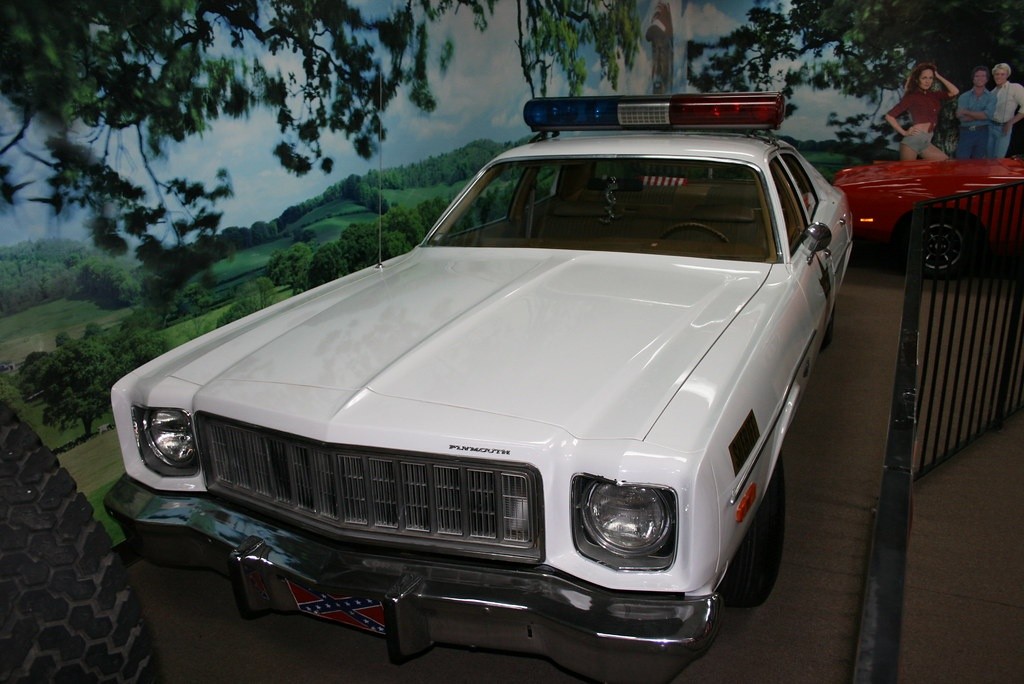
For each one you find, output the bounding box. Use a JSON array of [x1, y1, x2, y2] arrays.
[[987, 63, 1024, 158], [885, 63, 960, 161], [956, 66, 999, 160]]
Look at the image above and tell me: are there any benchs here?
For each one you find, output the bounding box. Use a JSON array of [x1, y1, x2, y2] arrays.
[[571, 183, 767, 224]]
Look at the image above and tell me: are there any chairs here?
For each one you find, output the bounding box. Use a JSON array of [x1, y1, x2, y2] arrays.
[[670, 180, 758, 247], [544, 179, 641, 239]]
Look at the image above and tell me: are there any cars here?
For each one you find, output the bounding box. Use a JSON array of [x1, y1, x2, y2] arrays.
[[103, 87, 853, 683], [827, 153, 1024, 276]]
